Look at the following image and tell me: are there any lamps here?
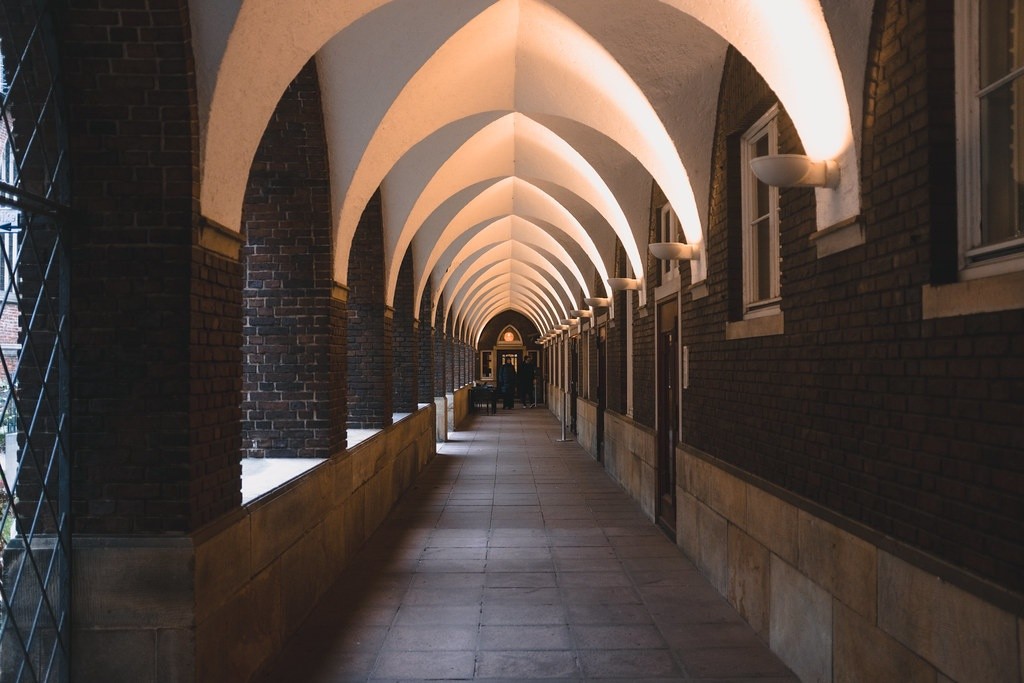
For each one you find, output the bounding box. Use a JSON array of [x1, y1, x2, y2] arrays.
[[561, 319, 577, 324], [570, 310, 591, 318], [540, 336, 550, 340], [537, 339, 545, 342], [554, 325, 568, 330], [545, 334, 556, 337], [584, 298, 611, 307], [548, 330, 562, 334], [748, 154, 839, 189], [607, 278, 642, 290], [535, 341, 544, 344], [648, 243, 700, 259]]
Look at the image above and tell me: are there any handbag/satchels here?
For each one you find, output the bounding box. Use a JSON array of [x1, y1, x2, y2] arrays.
[[514, 373, 520, 383]]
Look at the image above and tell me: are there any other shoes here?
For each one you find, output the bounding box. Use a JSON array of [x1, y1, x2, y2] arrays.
[[522, 405, 528, 408], [529, 403, 536, 408]]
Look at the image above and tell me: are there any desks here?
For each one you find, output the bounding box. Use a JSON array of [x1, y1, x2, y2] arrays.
[[471, 390, 496, 414]]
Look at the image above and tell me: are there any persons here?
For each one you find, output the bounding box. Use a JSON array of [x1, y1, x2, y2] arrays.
[[498, 356, 517, 410], [518, 356, 536, 408]]
